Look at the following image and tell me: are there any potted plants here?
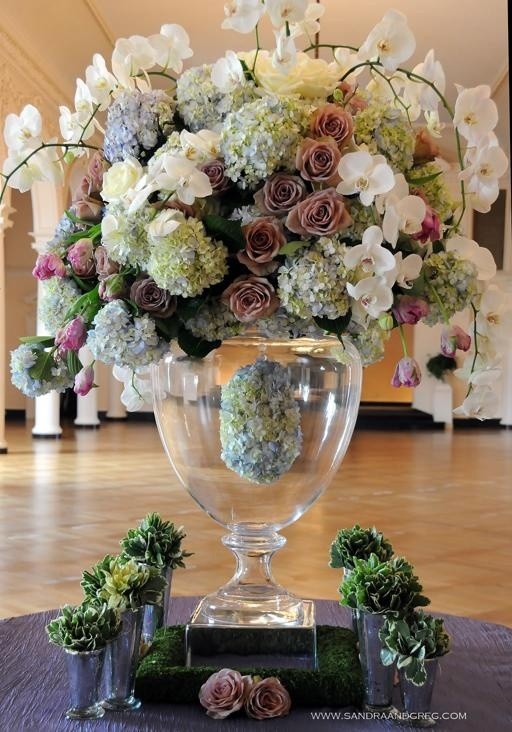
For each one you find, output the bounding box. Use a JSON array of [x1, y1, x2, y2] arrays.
[[328, 522, 449, 729], [44, 510, 194, 719]]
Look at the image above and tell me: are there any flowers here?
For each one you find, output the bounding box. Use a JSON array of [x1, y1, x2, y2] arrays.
[[199, 667, 291, 721], [0, 0, 512, 481]]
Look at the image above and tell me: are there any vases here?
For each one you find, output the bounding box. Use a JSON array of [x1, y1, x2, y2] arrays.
[[151, 335, 361, 671]]
